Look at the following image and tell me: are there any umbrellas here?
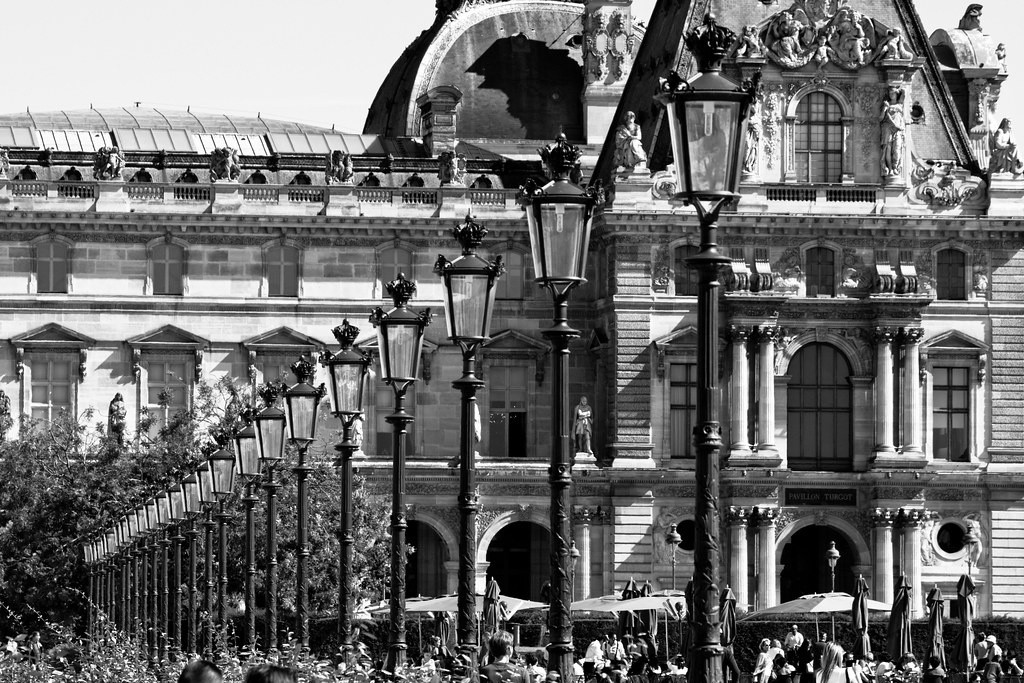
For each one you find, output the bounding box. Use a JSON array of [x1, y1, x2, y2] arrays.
[[923, 584, 948, 673], [741, 591, 917, 643], [571, 577, 745, 659], [365, 575, 550, 645], [852, 573, 871, 660], [956, 574, 977, 683], [887, 572, 912, 659]]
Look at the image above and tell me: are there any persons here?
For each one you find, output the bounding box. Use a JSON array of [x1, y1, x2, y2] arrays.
[[102, 147, 122, 177], [994, 118, 1018, 171], [175, 633, 1024, 683], [216, 149, 234, 180], [338, 152, 352, 181], [445, 150, 466, 183], [349, 414, 366, 445], [743, 0, 904, 70], [879, 86, 904, 175], [570, 396, 593, 454], [110, 393, 126, 444], [742, 105, 758, 172], [616, 111, 647, 166]]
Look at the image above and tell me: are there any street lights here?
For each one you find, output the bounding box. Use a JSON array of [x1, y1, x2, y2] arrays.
[[81, 426, 238, 676], [650, 10, 765, 683], [280, 353, 328, 663], [251, 381, 287, 654], [231, 401, 264, 663], [367, 271, 435, 683], [514, 128, 607, 683], [432, 204, 506, 683], [317, 317, 374, 677]]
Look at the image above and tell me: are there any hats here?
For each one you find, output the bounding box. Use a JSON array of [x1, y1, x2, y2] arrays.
[[985, 635, 997, 645]]
